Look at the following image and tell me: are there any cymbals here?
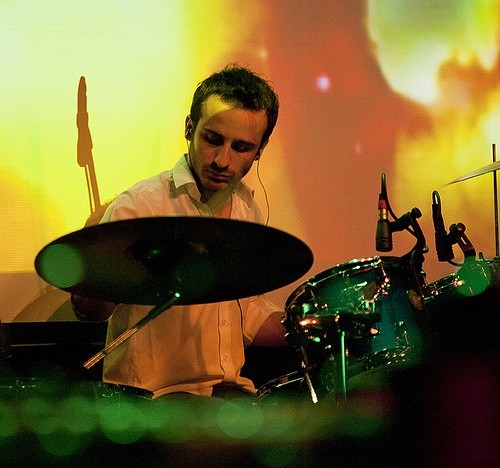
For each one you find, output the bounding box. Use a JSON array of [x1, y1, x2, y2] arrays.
[[33, 215, 316, 308], [441, 159, 500, 186]]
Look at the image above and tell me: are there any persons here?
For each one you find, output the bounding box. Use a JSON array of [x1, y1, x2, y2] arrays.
[[70, 67, 290, 399]]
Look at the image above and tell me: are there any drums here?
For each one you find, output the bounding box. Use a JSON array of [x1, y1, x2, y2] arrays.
[[279, 254, 443, 408]]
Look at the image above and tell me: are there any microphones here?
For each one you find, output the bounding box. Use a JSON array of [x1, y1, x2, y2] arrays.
[[74, 76, 94, 168], [432, 203, 454, 262], [375, 191, 394, 253]]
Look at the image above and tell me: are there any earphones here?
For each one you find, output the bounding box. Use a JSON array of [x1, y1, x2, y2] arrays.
[[185, 124, 193, 136], [257, 149, 261, 158]]
